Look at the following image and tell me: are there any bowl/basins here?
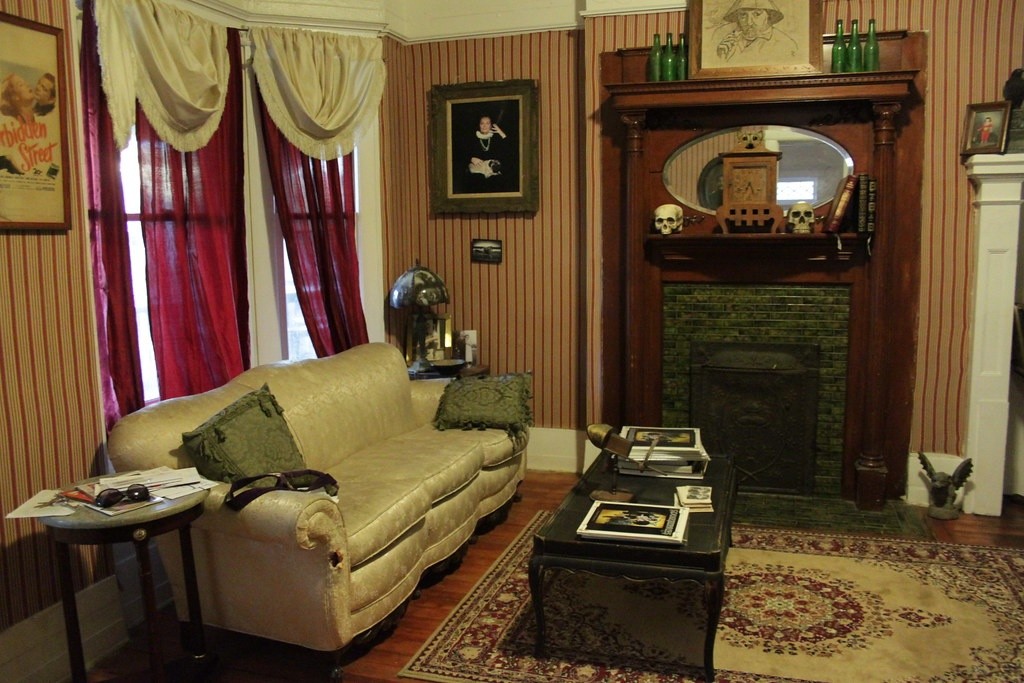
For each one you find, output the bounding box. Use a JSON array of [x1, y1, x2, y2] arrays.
[[429, 358, 468, 376]]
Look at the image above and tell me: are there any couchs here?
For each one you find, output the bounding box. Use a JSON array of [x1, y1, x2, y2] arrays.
[[109, 341, 528, 683]]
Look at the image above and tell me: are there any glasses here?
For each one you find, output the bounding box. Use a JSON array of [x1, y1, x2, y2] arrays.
[[96, 484, 150, 508]]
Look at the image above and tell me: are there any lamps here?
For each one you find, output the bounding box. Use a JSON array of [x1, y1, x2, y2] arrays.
[[387, 257, 450, 372]]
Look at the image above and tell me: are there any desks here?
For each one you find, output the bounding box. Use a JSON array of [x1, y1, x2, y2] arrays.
[[38, 470, 210, 683], [408, 362, 489, 380]]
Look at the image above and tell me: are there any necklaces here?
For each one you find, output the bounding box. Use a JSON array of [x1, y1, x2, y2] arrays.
[[479, 139, 491, 151]]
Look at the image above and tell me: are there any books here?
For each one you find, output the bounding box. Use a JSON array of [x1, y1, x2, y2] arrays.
[[576, 500, 690, 543], [822, 173, 877, 234], [673, 485, 715, 513], [618, 426, 711, 480], [57, 465, 202, 518]]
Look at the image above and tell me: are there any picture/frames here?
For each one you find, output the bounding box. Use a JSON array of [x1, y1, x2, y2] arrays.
[[960, 100, 1011, 157], [429, 79, 540, 215], [688, 0, 824, 80], [0, 11, 73, 232], [405, 312, 445, 367]]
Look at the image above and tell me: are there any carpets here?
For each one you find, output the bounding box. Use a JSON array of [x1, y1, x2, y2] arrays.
[[399, 508, 1024, 683]]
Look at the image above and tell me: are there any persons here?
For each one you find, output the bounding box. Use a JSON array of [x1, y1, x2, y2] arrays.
[[465, 115, 509, 193], [976, 116, 993, 145]]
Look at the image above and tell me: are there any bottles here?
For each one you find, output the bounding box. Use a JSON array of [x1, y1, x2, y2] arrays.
[[676, 33, 688, 81], [831, 19, 846, 73], [846, 19, 862, 72], [662, 33, 676, 81], [864, 19, 879, 71], [649, 33, 662, 82]]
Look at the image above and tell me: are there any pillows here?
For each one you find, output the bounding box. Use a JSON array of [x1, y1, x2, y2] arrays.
[[182, 382, 311, 489], [430, 369, 536, 437]]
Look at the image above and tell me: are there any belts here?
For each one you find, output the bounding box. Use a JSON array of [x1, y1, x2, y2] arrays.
[[225, 470, 339, 511]]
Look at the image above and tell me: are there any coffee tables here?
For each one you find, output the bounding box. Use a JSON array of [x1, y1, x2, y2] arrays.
[[529, 455, 739, 683]]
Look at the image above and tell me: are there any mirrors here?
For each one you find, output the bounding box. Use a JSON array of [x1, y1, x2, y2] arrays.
[[662, 125, 855, 217]]
